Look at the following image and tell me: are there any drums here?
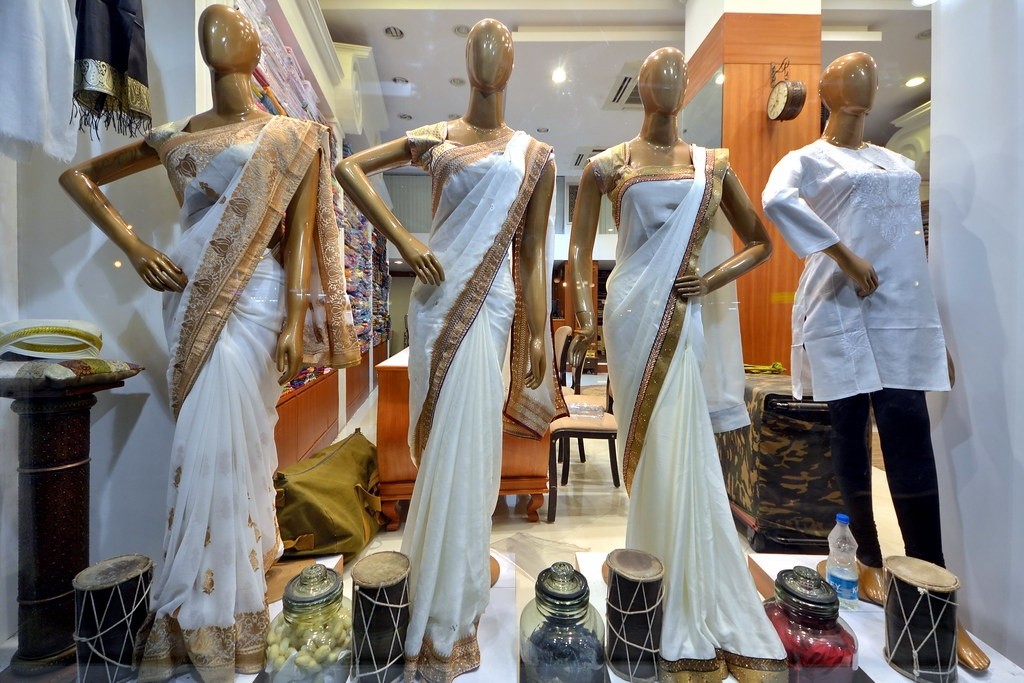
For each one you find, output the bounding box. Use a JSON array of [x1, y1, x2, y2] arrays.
[[350, 549, 412, 664], [71, 553, 156, 668], [882, 556, 963, 682], [603, 548, 666, 683]]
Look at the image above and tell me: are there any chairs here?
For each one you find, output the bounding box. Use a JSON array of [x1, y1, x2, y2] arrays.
[[547, 325, 619, 526]]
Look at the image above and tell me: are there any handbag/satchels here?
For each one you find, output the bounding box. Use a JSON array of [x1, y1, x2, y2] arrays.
[[272, 428, 382, 565]]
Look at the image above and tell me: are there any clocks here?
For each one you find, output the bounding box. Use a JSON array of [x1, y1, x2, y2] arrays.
[[766, 57, 807, 123]]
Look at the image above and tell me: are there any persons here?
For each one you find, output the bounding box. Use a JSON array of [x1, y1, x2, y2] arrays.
[[334, 18, 556, 683], [761, 51, 992, 672], [569, 46, 789, 683], [57, 4, 330, 683]]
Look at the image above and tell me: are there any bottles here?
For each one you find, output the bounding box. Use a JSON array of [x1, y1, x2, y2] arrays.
[[566, 403, 604, 418], [520, 562, 611, 683], [263, 563, 354, 683], [762, 566, 858, 683], [826, 514, 859, 611]]
